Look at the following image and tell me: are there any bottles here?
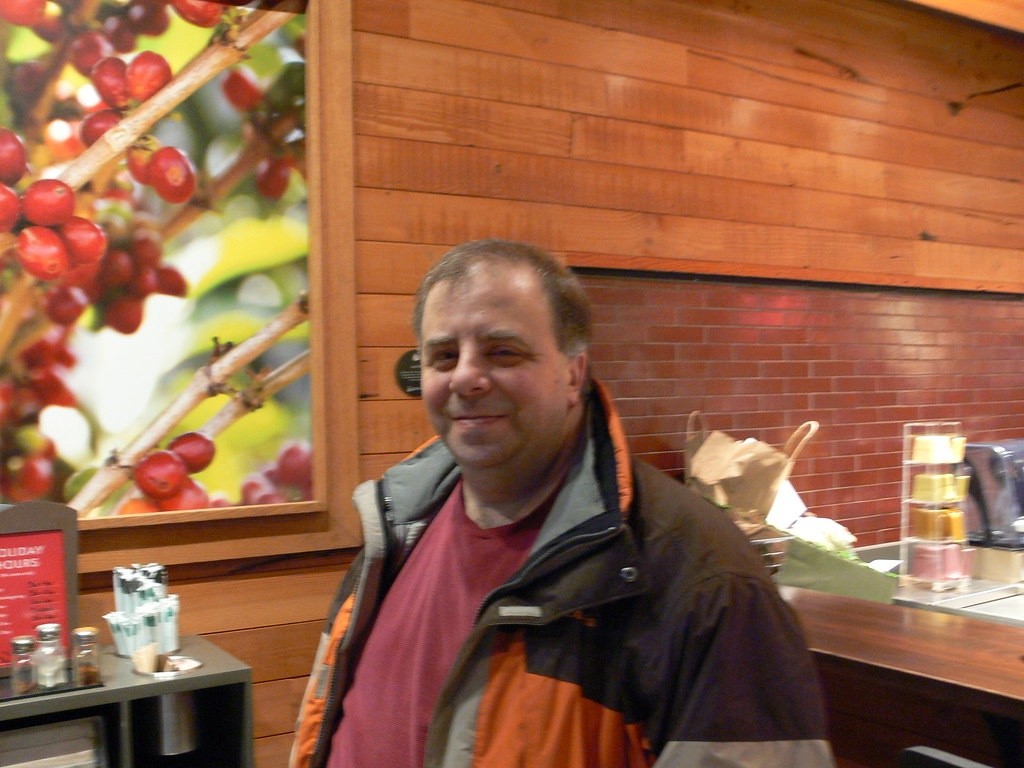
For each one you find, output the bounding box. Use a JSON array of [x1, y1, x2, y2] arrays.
[[10, 624, 100, 694]]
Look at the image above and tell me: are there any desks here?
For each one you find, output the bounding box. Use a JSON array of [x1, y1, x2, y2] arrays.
[[774, 583, 1024, 768], [855, 540, 1023, 625]]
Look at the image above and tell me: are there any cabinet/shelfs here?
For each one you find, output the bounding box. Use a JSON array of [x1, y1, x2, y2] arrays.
[[0, 632, 253, 767]]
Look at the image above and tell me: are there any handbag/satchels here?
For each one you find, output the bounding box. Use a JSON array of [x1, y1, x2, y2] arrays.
[[683, 402, 822, 526]]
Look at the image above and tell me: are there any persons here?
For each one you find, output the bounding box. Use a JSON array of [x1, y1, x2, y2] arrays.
[[289, 238, 833, 768]]
